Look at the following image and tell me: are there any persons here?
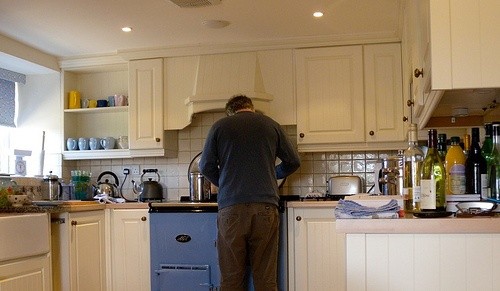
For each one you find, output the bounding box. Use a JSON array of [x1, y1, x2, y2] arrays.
[[198, 94, 301, 291]]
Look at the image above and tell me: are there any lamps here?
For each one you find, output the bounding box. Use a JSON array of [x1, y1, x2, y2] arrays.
[[452, 108, 469, 118]]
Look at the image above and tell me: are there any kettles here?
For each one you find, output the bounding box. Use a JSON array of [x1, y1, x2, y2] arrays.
[[131, 168, 164, 201]]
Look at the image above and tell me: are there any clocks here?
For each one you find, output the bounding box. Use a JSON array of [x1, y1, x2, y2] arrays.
[[15, 160, 26, 175]]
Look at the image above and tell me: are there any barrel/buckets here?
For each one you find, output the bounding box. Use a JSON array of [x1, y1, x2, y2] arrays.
[[188, 172, 212, 201]]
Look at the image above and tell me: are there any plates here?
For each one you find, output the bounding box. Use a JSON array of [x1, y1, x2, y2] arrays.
[[32, 200, 64, 206]]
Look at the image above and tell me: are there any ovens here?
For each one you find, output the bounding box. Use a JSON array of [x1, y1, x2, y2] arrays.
[[146, 200, 290, 291]]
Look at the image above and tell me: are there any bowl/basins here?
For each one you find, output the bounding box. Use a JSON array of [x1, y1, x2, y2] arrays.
[[7, 195, 27, 207]]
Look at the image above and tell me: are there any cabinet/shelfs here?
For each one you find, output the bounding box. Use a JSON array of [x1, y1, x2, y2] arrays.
[[402, 0, 500, 130], [107, 208, 151, 291], [0, 252, 53, 291], [59, 54, 130, 160], [116, 47, 178, 158], [296, 30, 412, 152], [51, 210, 108, 291], [288, 207, 337, 291]]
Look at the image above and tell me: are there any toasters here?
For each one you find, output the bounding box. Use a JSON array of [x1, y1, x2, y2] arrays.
[[326, 176, 366, 198]]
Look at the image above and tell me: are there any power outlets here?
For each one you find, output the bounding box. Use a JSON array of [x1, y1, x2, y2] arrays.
[[124, 164, 140, 175]]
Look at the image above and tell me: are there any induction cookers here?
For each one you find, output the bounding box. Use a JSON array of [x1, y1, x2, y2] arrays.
[[148, 154, 301, 209]]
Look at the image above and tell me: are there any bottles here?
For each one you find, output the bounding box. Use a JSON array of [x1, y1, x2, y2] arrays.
[[402, 120, 500, 214]]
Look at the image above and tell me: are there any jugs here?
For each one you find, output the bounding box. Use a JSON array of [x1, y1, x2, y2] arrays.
[[40, 170, 63, 201]]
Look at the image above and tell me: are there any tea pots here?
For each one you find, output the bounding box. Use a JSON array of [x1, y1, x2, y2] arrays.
[[68, 90, 82, 109], [91, 171, 120, 198]]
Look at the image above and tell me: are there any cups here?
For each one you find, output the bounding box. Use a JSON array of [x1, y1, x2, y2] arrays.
[[66, 137, 117, 150], [80, 95, 125, 108]]
